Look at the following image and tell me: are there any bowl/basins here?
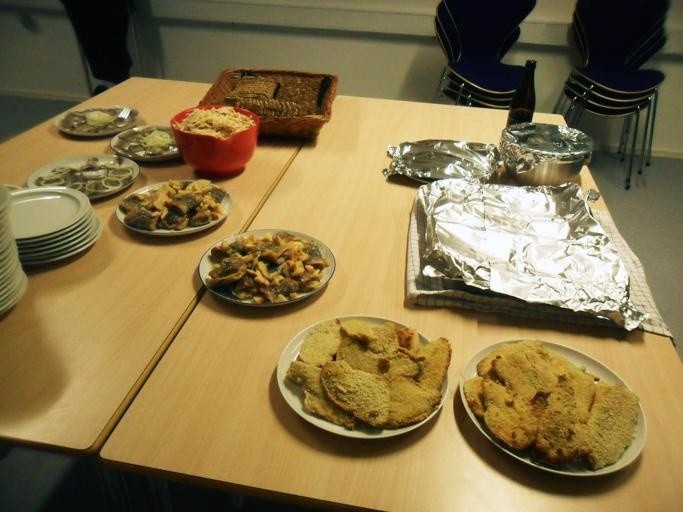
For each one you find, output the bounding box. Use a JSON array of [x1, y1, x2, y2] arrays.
[[501, 124, 587, 187], [172, 104, 261, 177]]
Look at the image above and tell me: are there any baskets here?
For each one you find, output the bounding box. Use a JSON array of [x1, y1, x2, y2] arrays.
[[198, 69, 338, 139]]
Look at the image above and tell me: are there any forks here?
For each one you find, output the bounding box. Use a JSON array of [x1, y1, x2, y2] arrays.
[[92, 108, 130, 133]]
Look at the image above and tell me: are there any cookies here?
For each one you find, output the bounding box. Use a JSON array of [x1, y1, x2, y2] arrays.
[[224, 75, 320, 116]]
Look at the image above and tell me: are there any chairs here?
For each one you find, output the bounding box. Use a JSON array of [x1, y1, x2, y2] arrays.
[[429, 1, 536, 110], [552, 0, 674, 192]]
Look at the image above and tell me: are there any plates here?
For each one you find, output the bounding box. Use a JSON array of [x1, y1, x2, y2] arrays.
[[111, 124, 182, 163], [9, 184, 102, 266], [459, 339, 648, 480], [114, 180, 233, 236], [30, 156, 138, 200], [276, 315, 446, 440], [60, 106, 141, 137], [197, 231, 337, 308], [0, 187, 30, 314]]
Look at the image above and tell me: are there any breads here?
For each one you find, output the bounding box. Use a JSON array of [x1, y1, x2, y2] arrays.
[[285, 318, 452, 432], [464, 338, 640, 470]]
